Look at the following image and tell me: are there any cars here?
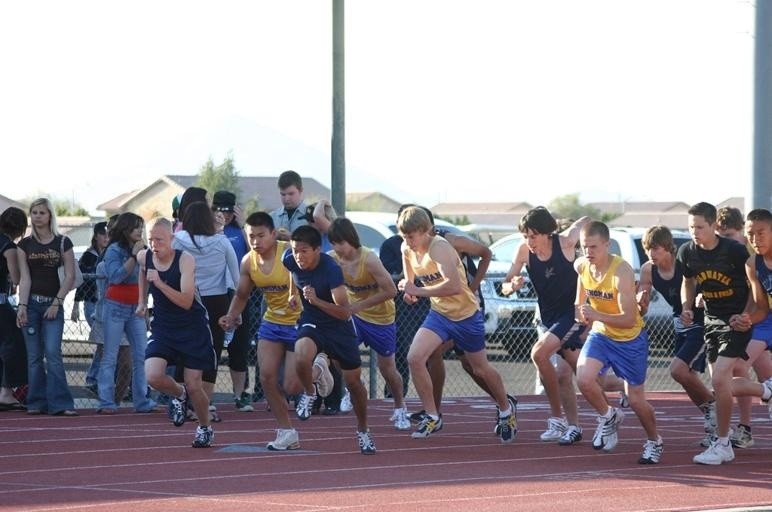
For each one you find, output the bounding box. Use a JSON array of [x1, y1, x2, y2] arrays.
[[7, 245, 229, 349]]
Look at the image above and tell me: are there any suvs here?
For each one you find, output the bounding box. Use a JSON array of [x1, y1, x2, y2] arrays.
[[486, 226, 692, 358], [343, 211, 537, 360]]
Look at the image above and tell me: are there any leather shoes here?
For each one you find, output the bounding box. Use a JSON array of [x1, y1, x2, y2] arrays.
[[150, 407, 166, 413], [58, 410, 80, 416], [100, 409, 114, 415], [2, 401, 26, 412], [27, 410, 41, 415]]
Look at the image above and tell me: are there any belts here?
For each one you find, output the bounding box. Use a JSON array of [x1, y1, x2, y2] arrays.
[[29, 293, 59, 303]]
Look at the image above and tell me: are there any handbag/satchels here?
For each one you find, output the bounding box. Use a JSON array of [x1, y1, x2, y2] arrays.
[[58, 235, 84, 291]]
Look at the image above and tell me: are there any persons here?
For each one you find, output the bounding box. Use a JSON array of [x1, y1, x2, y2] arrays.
[[675, 201, 771, 465], [0, 207, 29, 412], [382, 203, 425, 396], [70, 222, 104, 397], [297, 201, 340, 254], [95, 212, 169, 415], [573, 221, 664, 463], [209, 191, 250, 270], [395, 207, 520, 443], [134, 217, 216, 447], [325, 216, 411, 433], [635, 227, 717, 449], [263, 173, 311, 234], [733, 210, 772, 453], [500, 206, 593, 443], [14, 198, 79, 419], [171, 200, 245, 424], [281, 227, 379, 455], [216, 212, 335, 451], [420, 209, 514, 435], [713, 207, 755, 257]]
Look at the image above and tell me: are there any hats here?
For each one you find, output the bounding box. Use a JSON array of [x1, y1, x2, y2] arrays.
[[211, 191, 236, 212], [92, 222, 106, 239], [298, 202, 318, 222]]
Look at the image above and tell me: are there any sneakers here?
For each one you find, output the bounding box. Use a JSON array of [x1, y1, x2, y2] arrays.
[[693, 442, 737, 466], [540, 418, 569, 442], [394, 409, 411, 431], [411, 413, 444, 439], [702, 434, 721, 448], [236, 397, 255, 413], [558, 425, 583, 445], [338, 389, 355, 414], [405, 409, 445, 426], [731, 427, 758, 454], [322, 406, 337, 416], [601, 407, 625, 449], [193, 425, 215, 448], [313, 353, 334, 398], [499, 398, 518, 443], [295, 384, 317, 421], [702, 402, 720, 433], [172, 384, 188, 426], [639, 434, 663, 464], [187, 408, 198, 422], [209, 403, 222, 422], [355, 426, 378, 457], [592, 417, 607, 448], [766, 378, 772, 426], [267, 430, 301, 451]]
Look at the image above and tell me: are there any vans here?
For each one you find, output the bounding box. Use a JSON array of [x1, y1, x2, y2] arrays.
[[457, 223, 520, 244]]
